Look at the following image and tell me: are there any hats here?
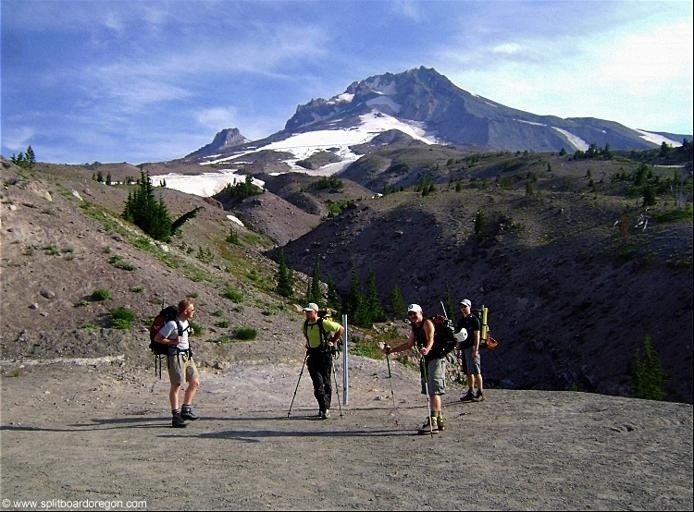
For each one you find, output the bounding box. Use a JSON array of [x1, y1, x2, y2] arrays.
[[178, 300, 191, 317], [407, 304, 422, 314], [303, 303, 319, 312], [460, 298, 472, 307]]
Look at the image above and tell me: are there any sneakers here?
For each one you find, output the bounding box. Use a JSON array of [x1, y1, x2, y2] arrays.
[[460, 390, 485, 401], [171, 416, 187, 427], [180, 411, 199, 420], [320, 409, 330, 418]]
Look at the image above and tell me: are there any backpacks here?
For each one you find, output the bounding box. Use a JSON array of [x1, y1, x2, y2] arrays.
[[150, 305, 191, 354], [413, 313, 457, 355], [472, 308, 490, 348], [303, 308, 337, 354]]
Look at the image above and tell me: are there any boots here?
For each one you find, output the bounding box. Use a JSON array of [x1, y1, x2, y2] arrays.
[[418, 415, 444, 435]]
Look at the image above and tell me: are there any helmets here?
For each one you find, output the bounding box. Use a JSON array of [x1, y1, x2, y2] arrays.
[[452, 328, 468, 342]]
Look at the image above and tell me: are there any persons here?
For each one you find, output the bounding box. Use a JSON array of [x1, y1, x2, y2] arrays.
[[456, 298, 485, 403], [154, 299, 199, 427], [303, 303, 345, 419], [381, 304, 445, 434]]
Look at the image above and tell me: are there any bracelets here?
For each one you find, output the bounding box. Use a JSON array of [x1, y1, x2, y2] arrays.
[[473, 348, 479, 352]]
